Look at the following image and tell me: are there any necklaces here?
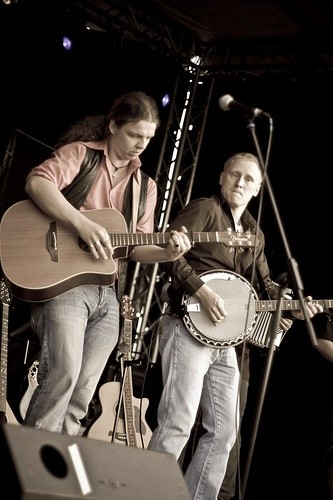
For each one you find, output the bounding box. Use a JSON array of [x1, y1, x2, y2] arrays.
[[110, 158, 131, 178]]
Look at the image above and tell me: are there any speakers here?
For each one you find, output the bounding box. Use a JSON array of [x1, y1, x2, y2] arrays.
[[0, 422, 192, 500]]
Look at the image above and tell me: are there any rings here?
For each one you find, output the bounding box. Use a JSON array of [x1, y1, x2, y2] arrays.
[[175, 244, 180, 248]]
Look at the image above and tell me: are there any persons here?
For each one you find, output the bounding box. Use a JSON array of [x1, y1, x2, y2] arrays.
[[146, 153, 324, 500], [24, 92, 192, 436]]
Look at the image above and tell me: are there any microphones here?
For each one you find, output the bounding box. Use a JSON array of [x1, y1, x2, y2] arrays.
[[218, 94, 264, 117]]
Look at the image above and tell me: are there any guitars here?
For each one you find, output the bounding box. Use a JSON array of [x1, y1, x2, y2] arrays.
[[86, 294, 153, 450], [0, 200, 259, 303]]
